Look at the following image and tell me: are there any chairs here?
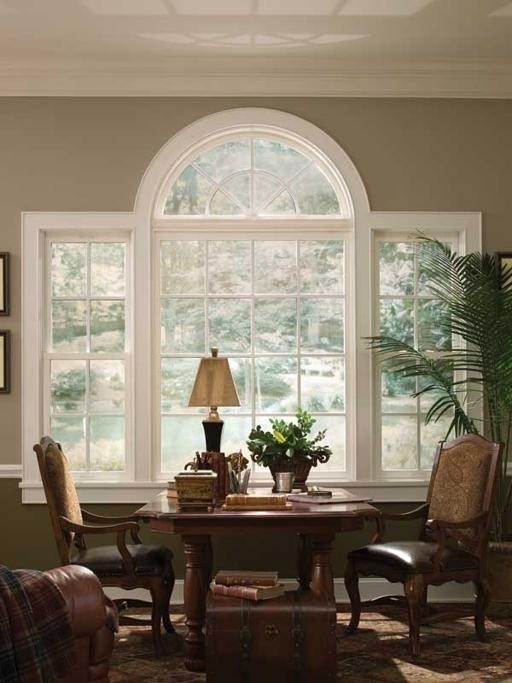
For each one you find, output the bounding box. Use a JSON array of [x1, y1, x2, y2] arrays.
[[344, 435, 504, 659], [31, 436, 177, 659]]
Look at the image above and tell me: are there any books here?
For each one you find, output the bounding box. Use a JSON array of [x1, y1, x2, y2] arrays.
[[221, 494, 293, 511], [209, 569, 300, 602]]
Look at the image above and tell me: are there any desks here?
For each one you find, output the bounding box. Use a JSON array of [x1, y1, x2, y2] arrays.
[[129, 488, 380, 673]]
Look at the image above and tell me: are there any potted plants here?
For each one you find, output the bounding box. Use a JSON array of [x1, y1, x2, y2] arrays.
[[361, 227, 512, 624], [246, 409, 334, 493]]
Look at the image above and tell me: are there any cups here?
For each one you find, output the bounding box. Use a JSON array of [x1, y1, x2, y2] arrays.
[[276, 472, 295, 492]]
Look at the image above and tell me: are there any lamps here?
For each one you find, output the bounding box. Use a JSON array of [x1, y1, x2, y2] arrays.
[[187, 347, 242, 453]]
